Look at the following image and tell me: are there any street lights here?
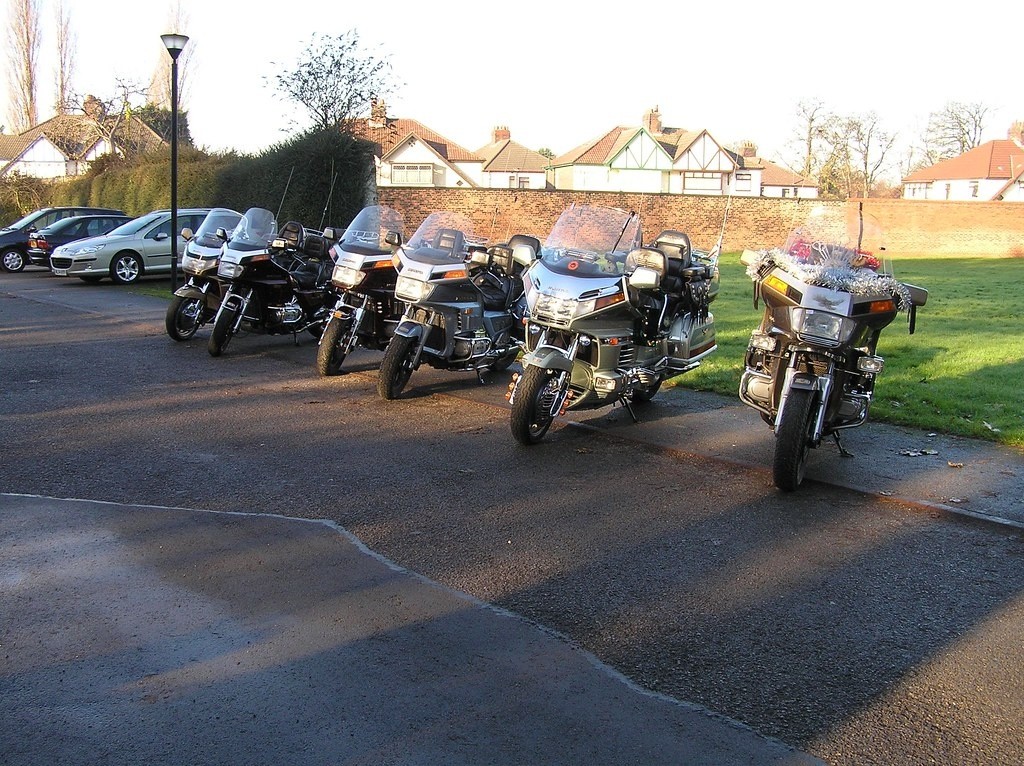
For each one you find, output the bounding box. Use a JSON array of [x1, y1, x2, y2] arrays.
[[161, 33, 189, 295]]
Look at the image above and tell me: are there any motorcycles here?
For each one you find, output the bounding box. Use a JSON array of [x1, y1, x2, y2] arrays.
[[318, 206, 489, 376], [166, 208, 323, 341], [505, 203, 720, 446], [378, 210, 542, 401], [739, 205, 929, 489], [208, 207, 380, 356]]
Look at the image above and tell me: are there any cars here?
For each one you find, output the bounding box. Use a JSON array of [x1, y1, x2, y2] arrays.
[[28, 214, 139, 267], [50, 208, 263, 285]]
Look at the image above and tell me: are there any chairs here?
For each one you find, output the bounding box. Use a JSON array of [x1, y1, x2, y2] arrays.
[[182, 220, 190, 229], [102, 224, 112, 234]]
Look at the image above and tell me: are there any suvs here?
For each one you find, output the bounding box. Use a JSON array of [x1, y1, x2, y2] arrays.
[[0, 206, 128, 273]]
